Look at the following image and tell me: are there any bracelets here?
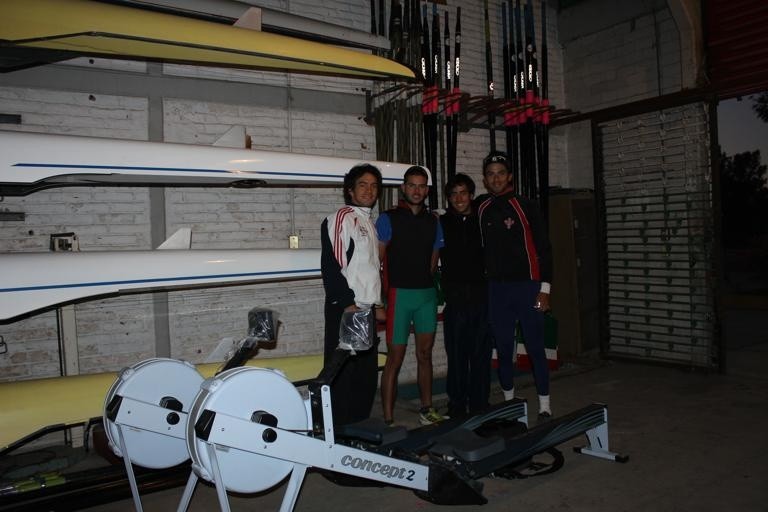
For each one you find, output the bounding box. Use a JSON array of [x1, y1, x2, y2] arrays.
[[539, 281, 552, 295]]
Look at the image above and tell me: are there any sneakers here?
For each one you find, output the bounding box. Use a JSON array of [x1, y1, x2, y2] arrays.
[[538, 410, 552, 424], [418, 407, 450, 426]]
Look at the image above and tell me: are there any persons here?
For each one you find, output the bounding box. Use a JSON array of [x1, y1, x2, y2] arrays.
[[439, 172, 493, 420], [375, 166, 445, 427], [320, 163, 383, 426], [472, 150, 554, 428]]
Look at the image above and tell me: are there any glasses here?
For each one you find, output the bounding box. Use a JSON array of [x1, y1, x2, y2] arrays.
[[484, 155, 507, 168]]
